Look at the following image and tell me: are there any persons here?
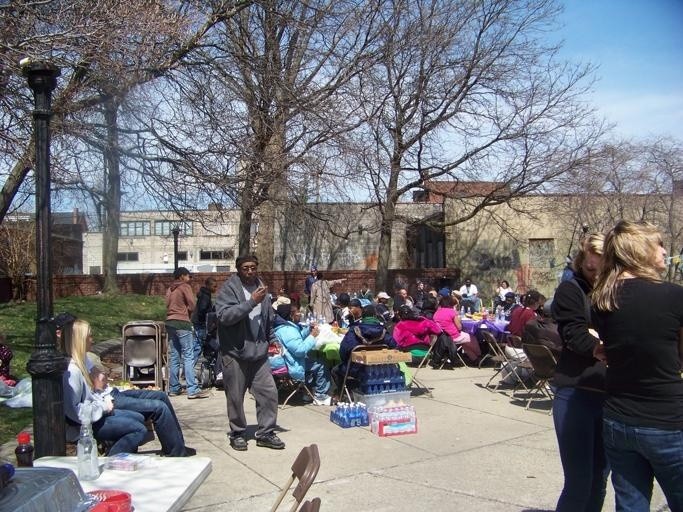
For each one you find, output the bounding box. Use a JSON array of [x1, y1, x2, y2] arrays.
[[58, 320, 148, 459], [53, 312, 198, 456], [162, 248, 561, 399], [212, 251, 287, 450], [550, 229, 613, 511], [597, 218, 682, 511]]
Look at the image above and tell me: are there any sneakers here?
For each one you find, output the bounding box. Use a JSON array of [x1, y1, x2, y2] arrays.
[[257, 433, 284, 448], [313, 397, 331, 405], [168, 388, 184, 396], [188, 392, 208, 399], [229, 436, 247, 451]]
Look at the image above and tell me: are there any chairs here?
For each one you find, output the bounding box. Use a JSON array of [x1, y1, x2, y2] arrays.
[[340, 343, 389, 404], [119, 319, 168, 391], [269, 334, 320, 410], [436, 321, 469, 370], [262, 442, 320, 511], [402, 333, 437, 398], [479, 330, 558, 415]]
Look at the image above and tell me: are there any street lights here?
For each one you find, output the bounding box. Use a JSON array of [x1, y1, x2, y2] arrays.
[[171, 224, 180, 273], [581, 222, 589, 239]]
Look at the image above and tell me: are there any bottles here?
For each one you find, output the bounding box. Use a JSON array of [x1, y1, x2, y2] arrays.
[[73, 418, 99, 481], [459, 305, 505, 324], [334, 400, 368, 427], [14, 430, 34, 469], [305, 311, 325, 327], [357, 365, 405, 394], [370, 405, 417, 436]]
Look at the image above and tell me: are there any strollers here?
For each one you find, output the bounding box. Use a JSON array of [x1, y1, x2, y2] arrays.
[[198, 310, 219, 389]]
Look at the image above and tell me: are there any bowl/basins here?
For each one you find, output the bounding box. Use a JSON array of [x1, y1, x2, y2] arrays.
[[81, 488, 131, 512]]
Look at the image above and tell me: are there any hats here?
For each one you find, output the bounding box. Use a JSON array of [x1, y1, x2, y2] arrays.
[[272, 296, 291, 310], [277, 305, 290, 318], [378, 292, 390, 299], [236, 256, 258, 267]]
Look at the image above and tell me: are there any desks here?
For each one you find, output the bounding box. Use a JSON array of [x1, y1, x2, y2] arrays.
[[297, 332, 413, 393], [31, 455, 214, 511]]
